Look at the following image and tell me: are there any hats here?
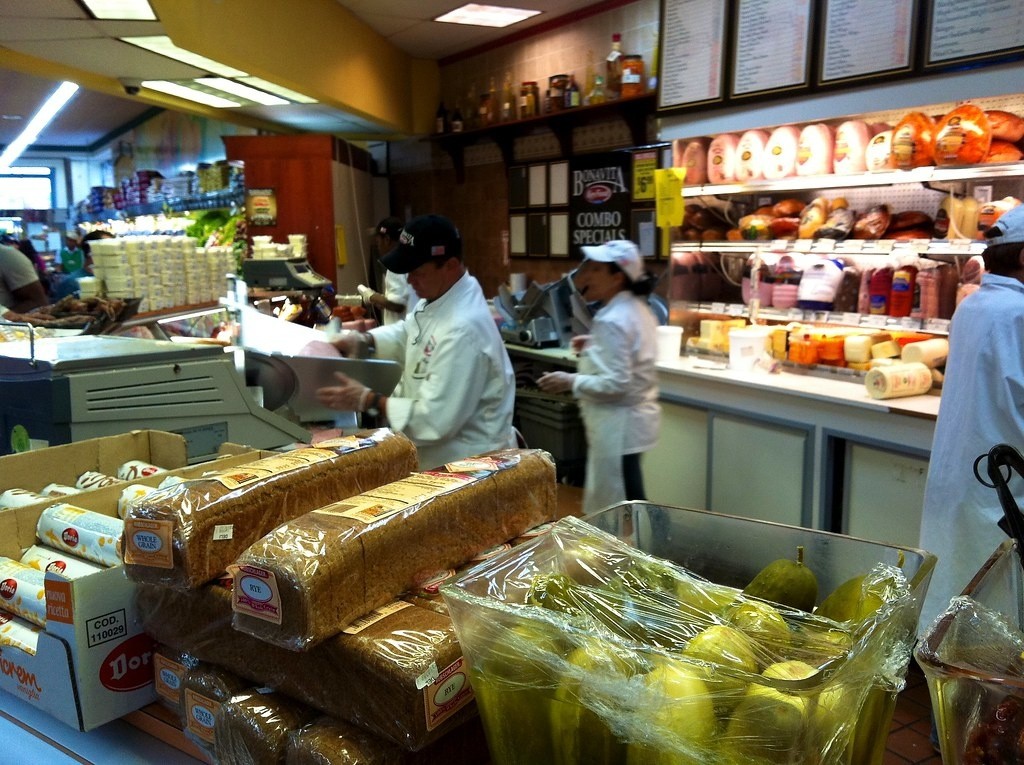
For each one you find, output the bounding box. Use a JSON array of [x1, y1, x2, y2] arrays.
[[386, 215, 463, 274], [66, 231, 78, 240], [376, 216, 408, 242], [580, 240, 644, 282], [986, 202, 1024, 247]]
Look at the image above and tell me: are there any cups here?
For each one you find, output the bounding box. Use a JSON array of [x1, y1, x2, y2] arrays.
[[941, 196, 977, 239], [788, 334, 842, 363], [657, 326, 683, 361], [509, 274, 527, 301]]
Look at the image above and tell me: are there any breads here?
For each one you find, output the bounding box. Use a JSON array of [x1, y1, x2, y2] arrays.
[[681, 104, 1024, 238], [116, 425, 559, 765]]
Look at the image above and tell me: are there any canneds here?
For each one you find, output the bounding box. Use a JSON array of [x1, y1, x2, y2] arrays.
[[619, 55, 644, 97], [519, 73, 569, 118]]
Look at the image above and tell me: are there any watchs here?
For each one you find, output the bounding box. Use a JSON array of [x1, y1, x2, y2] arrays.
[[367, 391, 384, 417]]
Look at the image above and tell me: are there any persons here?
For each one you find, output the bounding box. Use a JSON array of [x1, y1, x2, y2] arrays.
[[66, 230, 115, 278], [356, 216, 421, 328], [53, 232, 85, 273], [535, 240, 670, 553], [915, 204, 1023, 755], [0, 234, 81, 325], [311, 212, 516, 472]]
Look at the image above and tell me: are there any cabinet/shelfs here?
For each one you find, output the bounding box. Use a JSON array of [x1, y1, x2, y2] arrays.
[[672, 160, 1024, 336], [642, 391, 942, 609]]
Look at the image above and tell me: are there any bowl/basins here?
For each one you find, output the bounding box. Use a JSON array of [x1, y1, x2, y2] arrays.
[[75, 236, 238, 313], [742, 277, 799, 308], [250, 234, 307, 260], [737, 214, 771, 239]]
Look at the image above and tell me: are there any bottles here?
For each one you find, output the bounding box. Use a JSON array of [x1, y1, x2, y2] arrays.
[[544, 75, 581, 112], [581, 34, 658, 106], [858, 265, 959, 317], [435, 76, 539, 133]]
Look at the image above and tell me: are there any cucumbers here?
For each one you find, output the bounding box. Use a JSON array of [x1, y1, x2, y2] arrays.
[[478, 542, 910, 765]]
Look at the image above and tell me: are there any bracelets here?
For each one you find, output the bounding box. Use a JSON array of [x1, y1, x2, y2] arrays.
[[357, 387, 371, 412]]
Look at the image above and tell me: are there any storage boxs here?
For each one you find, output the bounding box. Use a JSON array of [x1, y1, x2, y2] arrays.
[[437, 500, 941, 765], [0, 429, 282, 733], [515, 395, 591, 483]]
